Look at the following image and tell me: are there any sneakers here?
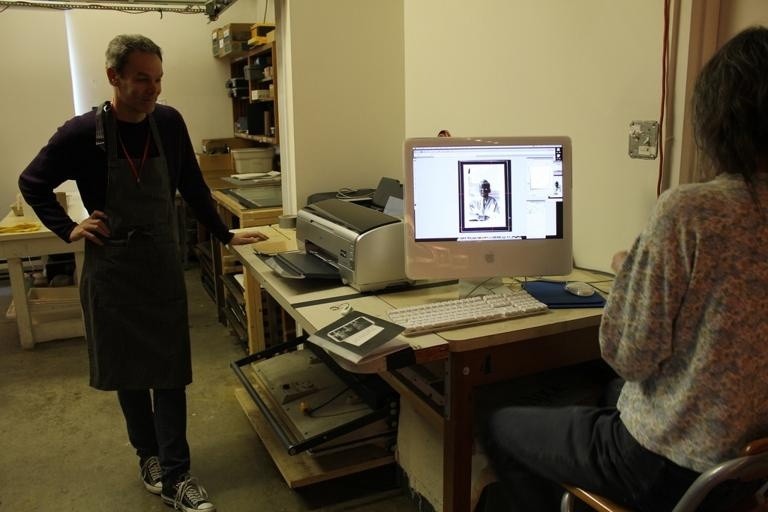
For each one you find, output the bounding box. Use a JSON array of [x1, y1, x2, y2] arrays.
[[161, 472, 216, 512], [140, 456, 163, 495]]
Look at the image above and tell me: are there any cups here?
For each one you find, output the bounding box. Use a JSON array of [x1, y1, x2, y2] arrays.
[[20, 194, 41, 224]]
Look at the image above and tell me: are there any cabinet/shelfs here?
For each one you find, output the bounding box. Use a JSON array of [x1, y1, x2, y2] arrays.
[[230, 42, 279, 144]]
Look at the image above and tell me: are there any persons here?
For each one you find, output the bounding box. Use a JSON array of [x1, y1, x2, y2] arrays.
[[474, 27, 767, 512], [18, 36, 269, 512], [475, 180, 497, 221]]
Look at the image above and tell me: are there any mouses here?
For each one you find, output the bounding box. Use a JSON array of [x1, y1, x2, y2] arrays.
[[564, 280, 595, 297]]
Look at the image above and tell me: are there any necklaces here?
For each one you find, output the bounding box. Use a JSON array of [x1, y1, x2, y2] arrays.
[[116, 129, 151, 183]]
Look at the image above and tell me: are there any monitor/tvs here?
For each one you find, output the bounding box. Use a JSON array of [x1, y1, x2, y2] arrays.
[[405, 136, 572, 276]]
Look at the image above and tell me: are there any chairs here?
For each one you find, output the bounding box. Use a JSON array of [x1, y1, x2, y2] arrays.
[[559, 433, 768, 511]]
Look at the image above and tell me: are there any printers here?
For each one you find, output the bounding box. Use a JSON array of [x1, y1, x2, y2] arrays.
[[253, 176, 404, 293]]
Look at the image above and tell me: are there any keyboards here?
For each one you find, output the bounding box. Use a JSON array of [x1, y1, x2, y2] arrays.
[[387, 290, 548, 336]]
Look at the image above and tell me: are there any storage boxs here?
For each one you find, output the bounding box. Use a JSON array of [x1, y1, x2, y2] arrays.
[[210, 22, 256, 59], [196, 152, 233, 195]]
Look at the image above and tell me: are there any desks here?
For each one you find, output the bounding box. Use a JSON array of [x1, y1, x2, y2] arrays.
[[223, 221, 618, 511], [0, 190, 88, 348], [211, 186, 283, 354]]
